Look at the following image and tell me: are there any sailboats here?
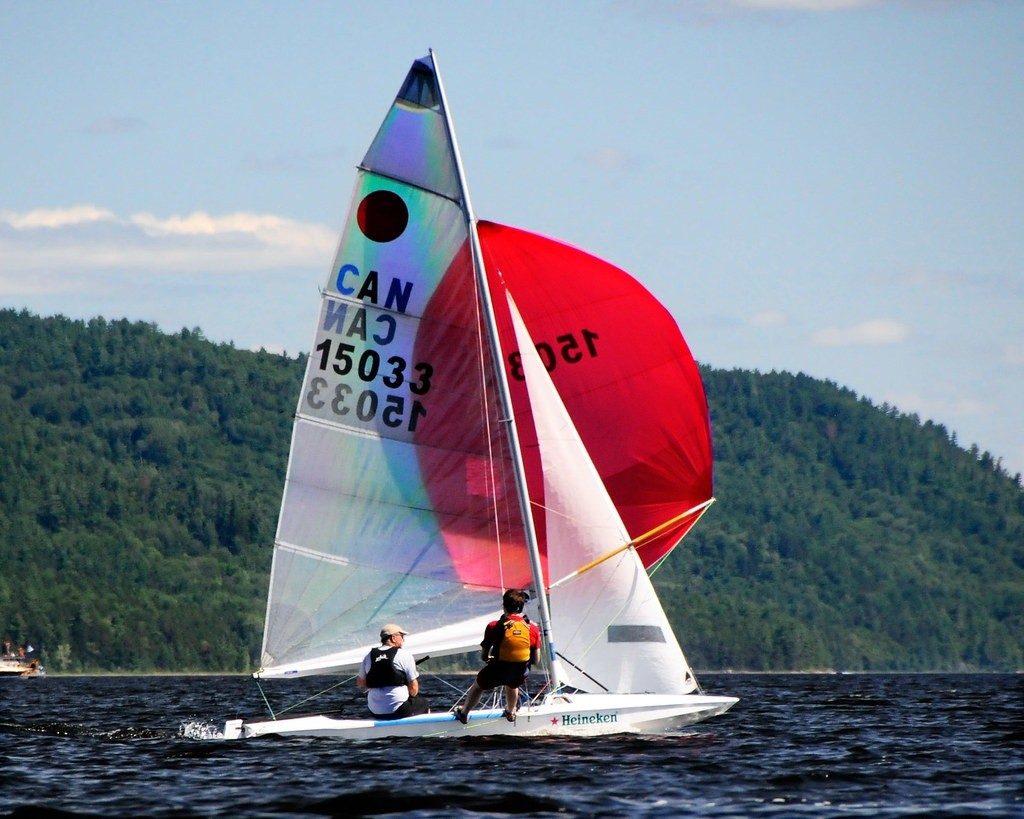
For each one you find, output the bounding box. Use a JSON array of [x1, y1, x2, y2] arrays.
[[224, 46, 739, 739]]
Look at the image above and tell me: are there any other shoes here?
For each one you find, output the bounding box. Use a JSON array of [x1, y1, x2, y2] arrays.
[[504, 705, 516, 721], [455, 706, 468, 723]]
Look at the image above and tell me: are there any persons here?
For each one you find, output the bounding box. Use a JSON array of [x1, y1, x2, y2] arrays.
[[454, 589, 542, 723], [359, 624, 428, 719]]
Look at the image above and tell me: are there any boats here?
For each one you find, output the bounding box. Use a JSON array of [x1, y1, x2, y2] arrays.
[[0, 644, 48, 678]]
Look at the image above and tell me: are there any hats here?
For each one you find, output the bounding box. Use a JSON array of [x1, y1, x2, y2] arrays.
[[380, 624, 407, 642]]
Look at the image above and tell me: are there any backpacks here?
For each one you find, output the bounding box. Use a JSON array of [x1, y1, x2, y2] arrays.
[[490, 615, 530, 662]]
